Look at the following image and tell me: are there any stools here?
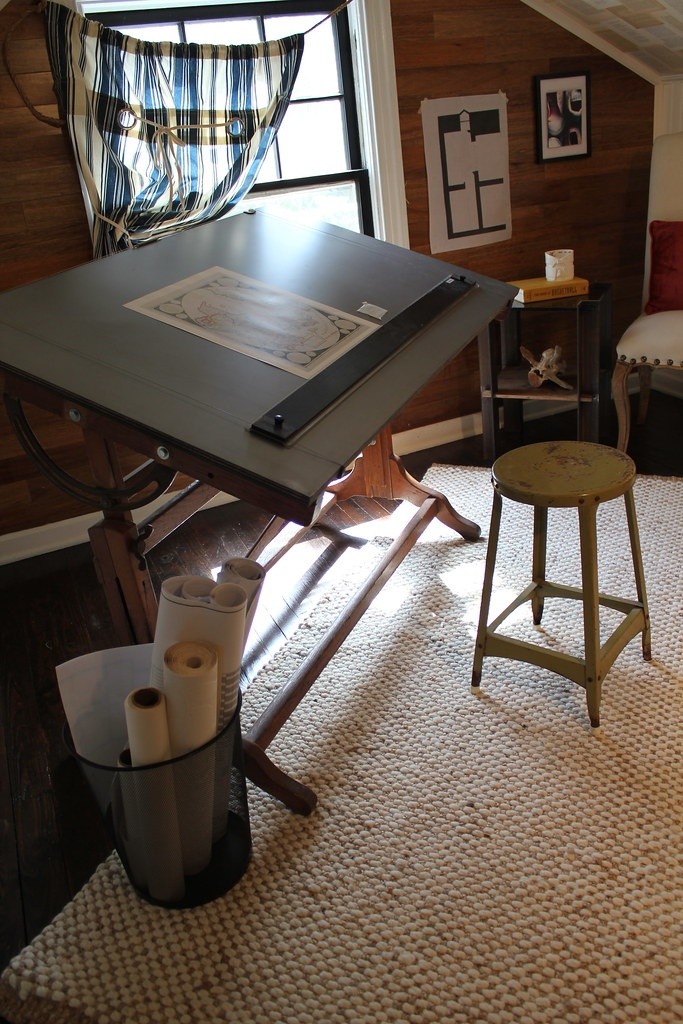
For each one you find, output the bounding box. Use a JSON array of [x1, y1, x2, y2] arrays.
[[471, 440, 652, 729]]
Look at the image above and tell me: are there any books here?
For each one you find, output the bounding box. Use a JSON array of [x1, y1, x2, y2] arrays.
[[505, 275, 589, 303]]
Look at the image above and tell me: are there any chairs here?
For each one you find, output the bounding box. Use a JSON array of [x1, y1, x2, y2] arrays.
[[613, 132, 683, 454]]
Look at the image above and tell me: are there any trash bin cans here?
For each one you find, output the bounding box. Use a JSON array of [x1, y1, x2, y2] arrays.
[[60, 685, 253, 909]]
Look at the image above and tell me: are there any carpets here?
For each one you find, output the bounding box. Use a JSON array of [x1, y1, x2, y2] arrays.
[[6, 464, 683, 1024]]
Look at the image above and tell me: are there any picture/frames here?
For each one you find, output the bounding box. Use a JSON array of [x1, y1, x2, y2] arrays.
[[532, 69, 589, 163]]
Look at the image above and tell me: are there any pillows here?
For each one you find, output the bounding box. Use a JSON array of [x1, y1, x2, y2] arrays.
[[644, 220, 683, 314]]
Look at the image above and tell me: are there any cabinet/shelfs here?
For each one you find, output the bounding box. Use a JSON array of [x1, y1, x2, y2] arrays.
[[477, 282, 612, 460]]
